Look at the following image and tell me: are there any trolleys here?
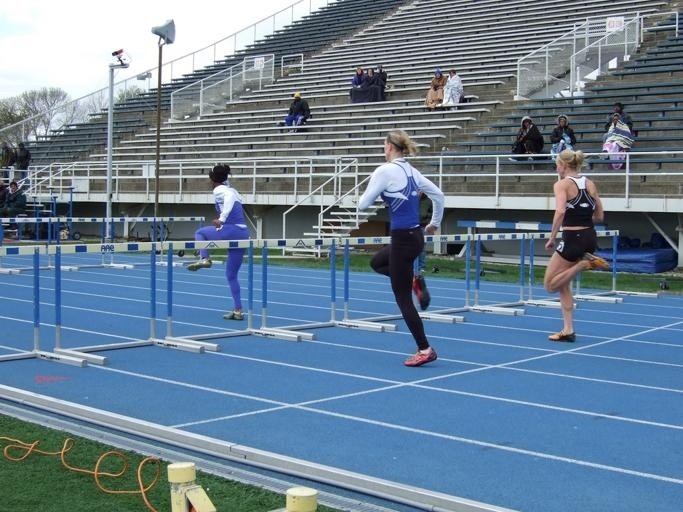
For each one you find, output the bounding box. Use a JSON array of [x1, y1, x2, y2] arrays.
[[36, 210, 82, 240]]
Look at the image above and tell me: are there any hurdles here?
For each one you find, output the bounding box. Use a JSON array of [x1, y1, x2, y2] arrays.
[[258, 237, 384, 340], [340, 234, 455, 331], [423, 234, 517, 321], [47, 241, 205, 365], [0, 216, 205, 252], [162, 239, 302, 352], [0, 246, 89, 368], [529, 230, 617, 309], [474, 233, 578, 315], [577, 230, 659, 302]]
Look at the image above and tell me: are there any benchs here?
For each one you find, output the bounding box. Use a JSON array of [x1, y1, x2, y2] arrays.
[[0, 0, 683, 183], [455, 218, 606, 267]]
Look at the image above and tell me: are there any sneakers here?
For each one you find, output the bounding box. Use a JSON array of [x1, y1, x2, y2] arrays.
[[412, 275, 430, 310], [548, 330, 576, 342], [582, 252, 610, 270], [223, 311, 244, 320], [404, 346, 437, 366], [185, 259, 212, 271]]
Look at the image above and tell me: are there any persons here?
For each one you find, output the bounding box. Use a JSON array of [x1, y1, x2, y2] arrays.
[[0, 142, 15, 182], [0, 182, 26, 229], [14, 143, 31, 179], [0, 182, 6, 207], [543, 149, 610, 342], [601, 113, 632, 160], [443, 70, 463, 110], [426, 68, 447, 105], [358, 131, 444, 366], [349, 67, 385, 103], [605, 103, 633, 130], [185, 164, 249, 320], [549, 114, 576, 161], [285, 93, 309, 132], [508, 116, 545, 170]]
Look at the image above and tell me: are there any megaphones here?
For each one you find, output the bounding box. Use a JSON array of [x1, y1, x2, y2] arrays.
[[150, 19, 175, 45]]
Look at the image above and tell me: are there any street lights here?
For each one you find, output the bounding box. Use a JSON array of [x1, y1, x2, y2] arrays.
[[151, 18, 176, 220]]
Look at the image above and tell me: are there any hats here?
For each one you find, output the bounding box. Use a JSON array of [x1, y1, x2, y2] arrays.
[[295, 93, 300, 97]]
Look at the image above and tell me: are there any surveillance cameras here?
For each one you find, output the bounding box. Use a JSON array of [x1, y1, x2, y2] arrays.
[[112, 47, 123, 57]]
